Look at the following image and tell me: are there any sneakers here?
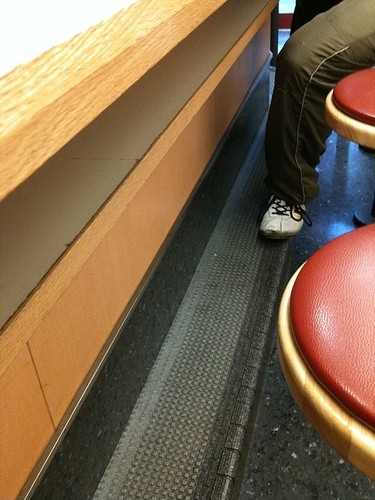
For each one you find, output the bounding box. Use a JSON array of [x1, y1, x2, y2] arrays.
[[260, 191, 312, 239]]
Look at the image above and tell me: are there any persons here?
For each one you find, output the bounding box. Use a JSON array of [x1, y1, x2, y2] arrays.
[[259, 0, 375, 240]]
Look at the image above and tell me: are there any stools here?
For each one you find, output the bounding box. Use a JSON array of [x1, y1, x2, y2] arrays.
[[277, 223, 375, 480], [325, 66, 375, 228]]
